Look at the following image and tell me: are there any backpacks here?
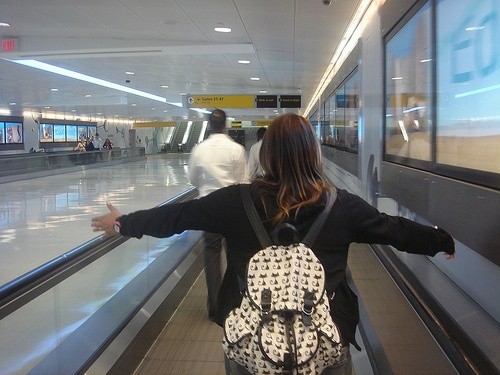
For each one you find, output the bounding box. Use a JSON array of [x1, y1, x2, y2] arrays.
[[223, 184, 343, 375]]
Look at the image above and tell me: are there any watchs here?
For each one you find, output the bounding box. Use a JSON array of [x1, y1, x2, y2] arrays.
[[112, 217, 121, 234]]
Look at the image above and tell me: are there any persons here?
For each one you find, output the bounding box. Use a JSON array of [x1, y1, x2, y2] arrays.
[[248, 124, 270, 182], [187, 109, 249, 322], [91, 113, 456, 374], [72, 133, 114, 167]]
